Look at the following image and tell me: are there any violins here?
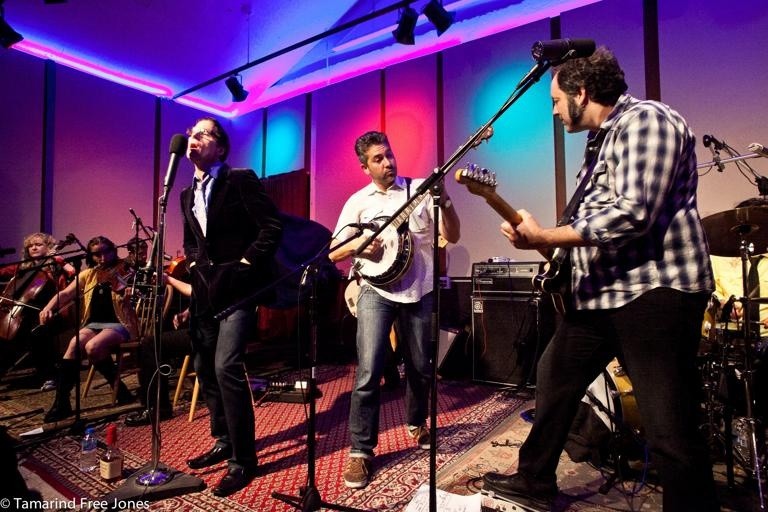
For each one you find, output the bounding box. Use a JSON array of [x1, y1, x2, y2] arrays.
[[96, 260, 142, 298], [167, 255, 188, 278]]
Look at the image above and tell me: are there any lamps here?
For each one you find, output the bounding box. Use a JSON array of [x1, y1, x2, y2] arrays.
[[167, 0, 455, 104]]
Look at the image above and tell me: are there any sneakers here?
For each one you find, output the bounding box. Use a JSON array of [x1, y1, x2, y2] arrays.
[[409, 427, 431, 450], [41, 380, 55, 391], [343, 457, 370, 487]]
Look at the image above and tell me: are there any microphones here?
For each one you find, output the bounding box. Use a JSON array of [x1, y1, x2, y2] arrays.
[[534, 36, 597, 64], [747, 142, 768, 156], [347, 218, 384, 231], [163, 129, 186, 188]]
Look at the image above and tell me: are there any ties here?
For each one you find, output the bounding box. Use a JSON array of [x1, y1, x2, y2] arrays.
[[195, 175, 210, 237]]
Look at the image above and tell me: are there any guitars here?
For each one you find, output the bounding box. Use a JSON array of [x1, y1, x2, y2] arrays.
[[453, 159, 574, 321]]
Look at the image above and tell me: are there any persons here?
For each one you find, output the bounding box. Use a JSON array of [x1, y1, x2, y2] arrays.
[[703, 195, 768, 340], [329, 129, 460, 490], [484, 42, 715, 512], [38, 237, 138, 423], [1, 233, 74, 376], [126, 272, 191, 428], [125, 239, 148, 268], [175, 116, 287, 497]]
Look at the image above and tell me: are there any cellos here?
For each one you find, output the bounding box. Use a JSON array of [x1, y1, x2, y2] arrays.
[[0, 232, 77, 340]]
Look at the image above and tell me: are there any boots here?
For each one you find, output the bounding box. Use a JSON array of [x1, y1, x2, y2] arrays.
[[94, 355, 131, 404], [44, 359, 75, 420]]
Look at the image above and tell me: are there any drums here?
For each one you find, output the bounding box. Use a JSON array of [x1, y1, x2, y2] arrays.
[[586, 360, 641, 436]]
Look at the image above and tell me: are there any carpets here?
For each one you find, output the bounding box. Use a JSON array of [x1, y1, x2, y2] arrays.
[[0, 367, 195, 449], [329, 387, 768, 512], [17, 363, 525, 512]]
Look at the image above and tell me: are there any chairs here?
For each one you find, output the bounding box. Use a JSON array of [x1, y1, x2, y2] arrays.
[[172, 350, 206, 424], [80, 283, 174, 406]]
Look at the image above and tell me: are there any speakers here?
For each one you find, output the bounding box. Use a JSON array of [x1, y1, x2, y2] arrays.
[[470, 298, 545, 393]]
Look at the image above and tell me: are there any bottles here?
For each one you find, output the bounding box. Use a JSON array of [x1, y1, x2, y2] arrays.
[[80, 423, 123, 483]]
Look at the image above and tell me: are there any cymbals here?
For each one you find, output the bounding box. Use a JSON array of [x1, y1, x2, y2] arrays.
[[701, 203, 766, 258]]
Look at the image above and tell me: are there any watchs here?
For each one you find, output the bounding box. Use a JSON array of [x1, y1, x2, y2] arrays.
[[441, 197, 452, 210]]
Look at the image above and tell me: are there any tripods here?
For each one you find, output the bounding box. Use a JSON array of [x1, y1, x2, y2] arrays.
[[212, 231, 377, 512], [19, 237, 151, 452]]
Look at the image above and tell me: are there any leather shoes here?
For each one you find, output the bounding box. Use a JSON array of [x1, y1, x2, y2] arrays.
[[187, 444, 226, 468], [213, 467, 250, 495], [125, 404, 172, 426], [482, 472, 559, 510]]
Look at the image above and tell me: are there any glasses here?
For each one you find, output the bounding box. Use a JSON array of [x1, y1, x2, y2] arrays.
[[185, 127, 219, 141]]
